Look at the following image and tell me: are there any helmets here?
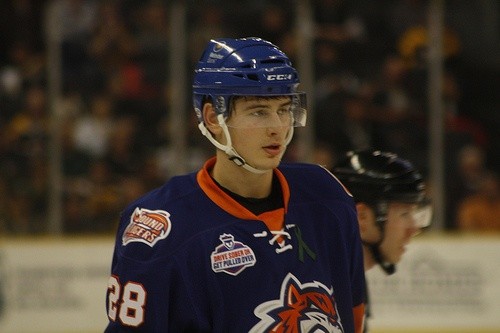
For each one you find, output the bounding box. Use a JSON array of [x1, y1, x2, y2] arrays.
[[193, 36, 301, 125], [330, 150, 432, 207]]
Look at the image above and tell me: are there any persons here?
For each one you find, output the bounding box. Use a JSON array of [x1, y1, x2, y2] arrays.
[[328, 149, 426, 275], [103, 36, 371, 333], [0, 1, 500, 228]]
